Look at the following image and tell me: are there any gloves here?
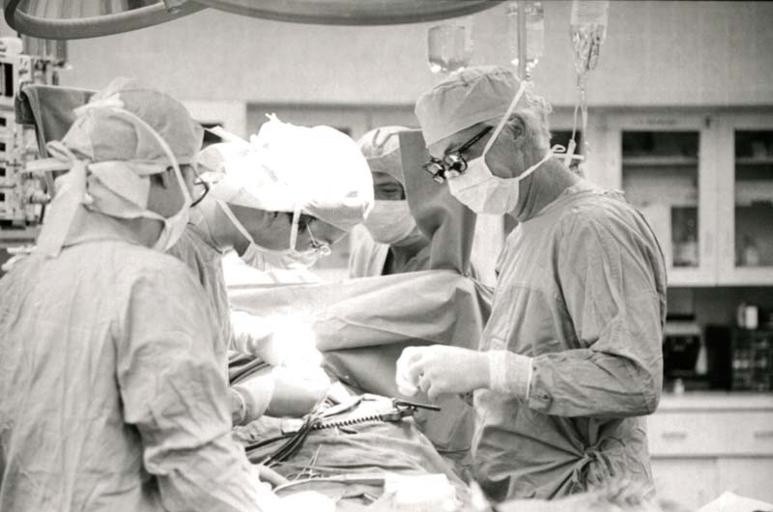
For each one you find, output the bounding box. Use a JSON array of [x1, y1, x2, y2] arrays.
[[394, 344, 532, 402]]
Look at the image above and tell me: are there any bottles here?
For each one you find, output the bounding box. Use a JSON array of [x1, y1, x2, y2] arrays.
[[570, 1, 613, 70], [506, 2, 546, 79], [423, 15, 476, 75]]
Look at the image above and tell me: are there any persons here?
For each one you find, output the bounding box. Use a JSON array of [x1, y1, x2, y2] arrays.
[[349, 126, 434, 277], [0, 77, 304, 512], [166, 111, 376, 428], [395, 65, 667, 511]]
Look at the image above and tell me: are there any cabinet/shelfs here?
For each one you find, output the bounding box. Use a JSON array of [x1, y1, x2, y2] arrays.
[[648, 407, 772, 510]]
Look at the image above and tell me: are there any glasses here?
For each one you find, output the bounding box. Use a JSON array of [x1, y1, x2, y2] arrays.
[[421, 125, 493, 183], [302, 216, 331, 260], [188, 163, 209, 207]]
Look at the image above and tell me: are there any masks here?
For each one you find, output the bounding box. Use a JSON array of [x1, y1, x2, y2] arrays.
[[447, 156, 519, 216], [153, 197, 193, 253], [362, 198, 416, 245], [240, 241, 318, 274]]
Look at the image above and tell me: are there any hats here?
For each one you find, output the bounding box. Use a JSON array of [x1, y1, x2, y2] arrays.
[[301, 125, 373, 233], [63, 76, 203, 166], [358, 125, 411, 199], [413, 64, 529, 149]]
